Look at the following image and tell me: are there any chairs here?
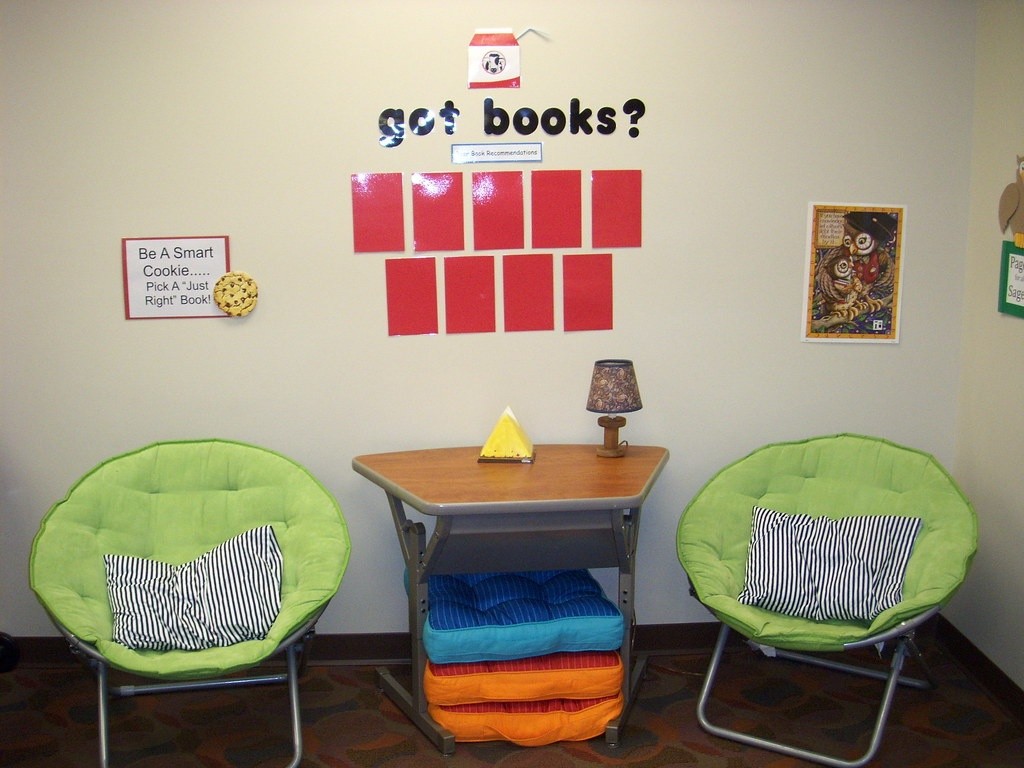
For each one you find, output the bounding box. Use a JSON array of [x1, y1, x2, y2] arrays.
[[676, 433, 977, 768], [29, 439, 352, 768]]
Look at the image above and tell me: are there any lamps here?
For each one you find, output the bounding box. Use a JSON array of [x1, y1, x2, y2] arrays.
[[586, 359, 642, 457]]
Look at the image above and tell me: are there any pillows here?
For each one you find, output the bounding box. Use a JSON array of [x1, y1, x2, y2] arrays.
[[102, 525, 283, 652], [736, 505, 923, 621]]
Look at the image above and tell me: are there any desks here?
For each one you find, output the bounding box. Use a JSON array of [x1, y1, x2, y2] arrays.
[[352, 444, 671, 755]]
